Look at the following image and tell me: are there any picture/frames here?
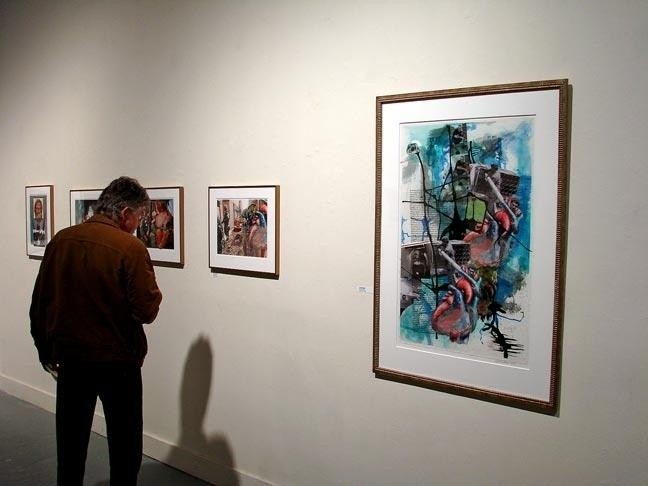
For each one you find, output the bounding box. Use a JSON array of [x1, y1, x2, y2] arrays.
[[23, 184, 282, 282], [372, 78, 573, 417]]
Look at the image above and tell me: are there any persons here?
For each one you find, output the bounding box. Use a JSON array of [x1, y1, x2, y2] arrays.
[[29, 177, 162, 486], [153, 200, 174, 249], [33, 200, 46, 244]]
